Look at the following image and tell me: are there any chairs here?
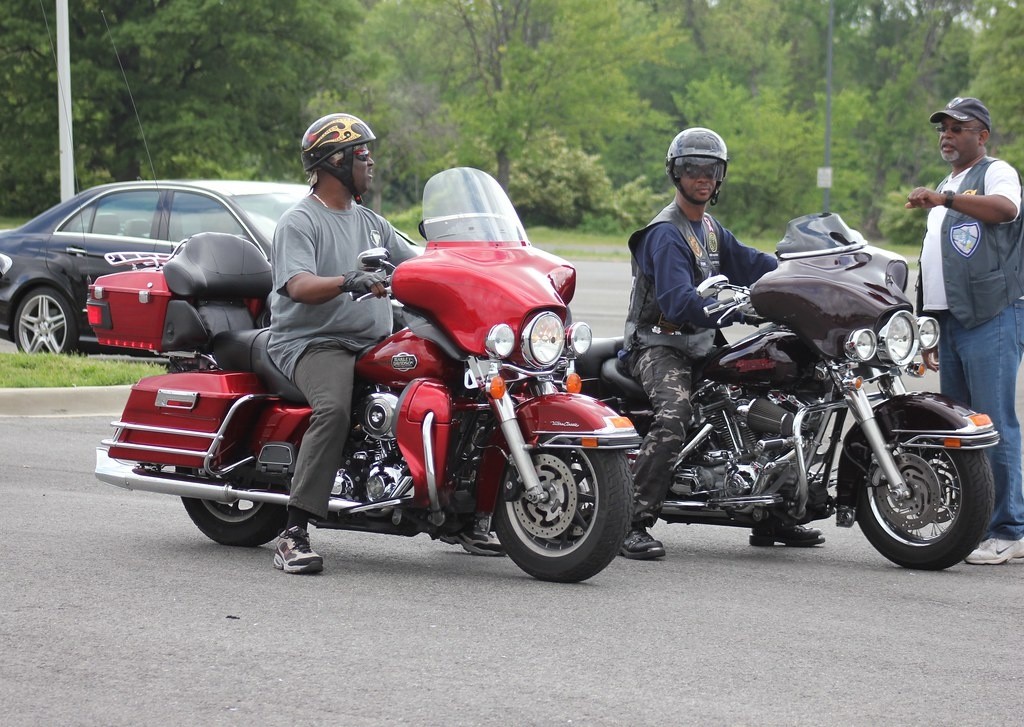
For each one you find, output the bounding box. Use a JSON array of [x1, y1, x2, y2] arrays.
[[159, 231, 274, 373], [91, 215, 152, 238]]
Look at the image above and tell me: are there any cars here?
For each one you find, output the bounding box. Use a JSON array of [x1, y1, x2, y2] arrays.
[[0, 179, 426, 357]]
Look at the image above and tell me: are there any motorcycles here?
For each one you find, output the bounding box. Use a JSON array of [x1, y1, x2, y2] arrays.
[[574, 211, 1000, 571], [86, 167, 645, 585]]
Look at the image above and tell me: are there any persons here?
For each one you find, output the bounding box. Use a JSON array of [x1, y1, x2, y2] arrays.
[[265, 113, 508, 573], [904, 97, 1024, 565], [617, 127, 825, 560]]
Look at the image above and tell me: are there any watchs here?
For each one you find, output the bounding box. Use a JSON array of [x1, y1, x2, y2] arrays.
[[943, 190, 955, 209]]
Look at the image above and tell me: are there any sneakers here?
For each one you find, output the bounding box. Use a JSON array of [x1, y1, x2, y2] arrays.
[[273, 526, 324, 573], [964, 536, 1024, 565], [439, 530, 506, 557]]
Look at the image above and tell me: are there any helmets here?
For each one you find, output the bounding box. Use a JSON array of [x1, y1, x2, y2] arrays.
[[665, 127, 729, 185], [300, 113, 376, 171]]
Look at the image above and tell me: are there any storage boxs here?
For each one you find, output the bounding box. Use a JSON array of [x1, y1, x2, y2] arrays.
[[86, 266, 174, 353]]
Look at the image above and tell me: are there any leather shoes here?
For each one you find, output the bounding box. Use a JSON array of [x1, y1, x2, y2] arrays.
[[749, 522, 826, 547], [618, 524, 665, 560]]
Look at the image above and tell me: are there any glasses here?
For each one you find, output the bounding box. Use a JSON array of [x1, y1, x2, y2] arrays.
[[935, 124, 983, 135], [685, 164, 718, 179], [353, 150, 371, 160]]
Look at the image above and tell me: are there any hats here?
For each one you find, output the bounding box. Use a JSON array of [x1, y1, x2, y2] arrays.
[[929, 97, 991, 134]]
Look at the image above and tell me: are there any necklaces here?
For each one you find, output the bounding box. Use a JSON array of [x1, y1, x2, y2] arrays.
[[313, 194, 329, 209]]
